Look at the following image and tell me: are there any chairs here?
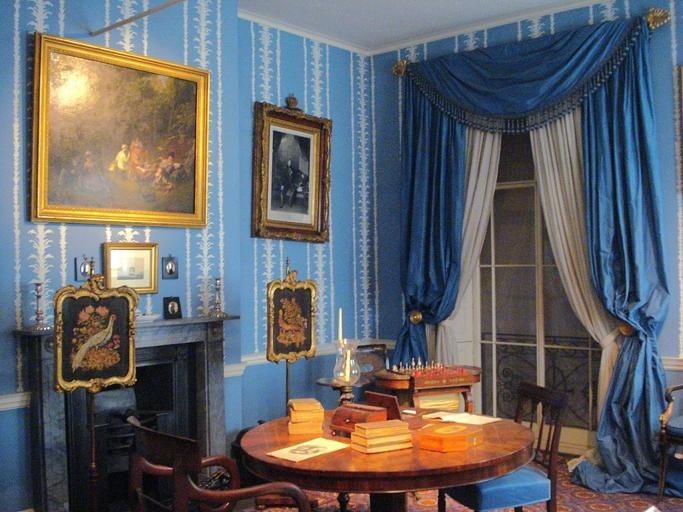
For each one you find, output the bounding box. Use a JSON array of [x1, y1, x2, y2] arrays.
[[126, 416, 312, 512], [436, 381, 569, 511], [656, 384, 683, 501]]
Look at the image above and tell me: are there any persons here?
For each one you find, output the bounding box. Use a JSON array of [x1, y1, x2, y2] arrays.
[[46, 135, 194, 206], [280, 160, 299, 208]]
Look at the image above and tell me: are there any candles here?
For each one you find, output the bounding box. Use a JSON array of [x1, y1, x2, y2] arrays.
[[342, 349, 351, 383], [338, 307, 343, 342]]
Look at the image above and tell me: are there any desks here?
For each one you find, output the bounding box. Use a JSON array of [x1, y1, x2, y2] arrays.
[[368, 365, 480, 414], [239, 409, 537, 511]]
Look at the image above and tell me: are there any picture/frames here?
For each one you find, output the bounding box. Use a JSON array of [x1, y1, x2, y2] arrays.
[[162, 256, 179, 280], [75, 255, 94, 282], [164, 297, 181, 319], [101, 242, 158, 294], [52, 274, 139, 394], [251, 95, 333, 244], [266, 269, 319, 365], [30, 30, 211, 229]]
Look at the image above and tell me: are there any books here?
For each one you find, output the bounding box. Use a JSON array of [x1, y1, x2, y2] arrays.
[[355, 419, 409, 435], [288, 408, 325, 422], [285, 417, 324, 435], [291, 397, 322, 410], [352, 442, 415, 454], [352, 429, 410, 446], [421, 411, 503, 427]]
